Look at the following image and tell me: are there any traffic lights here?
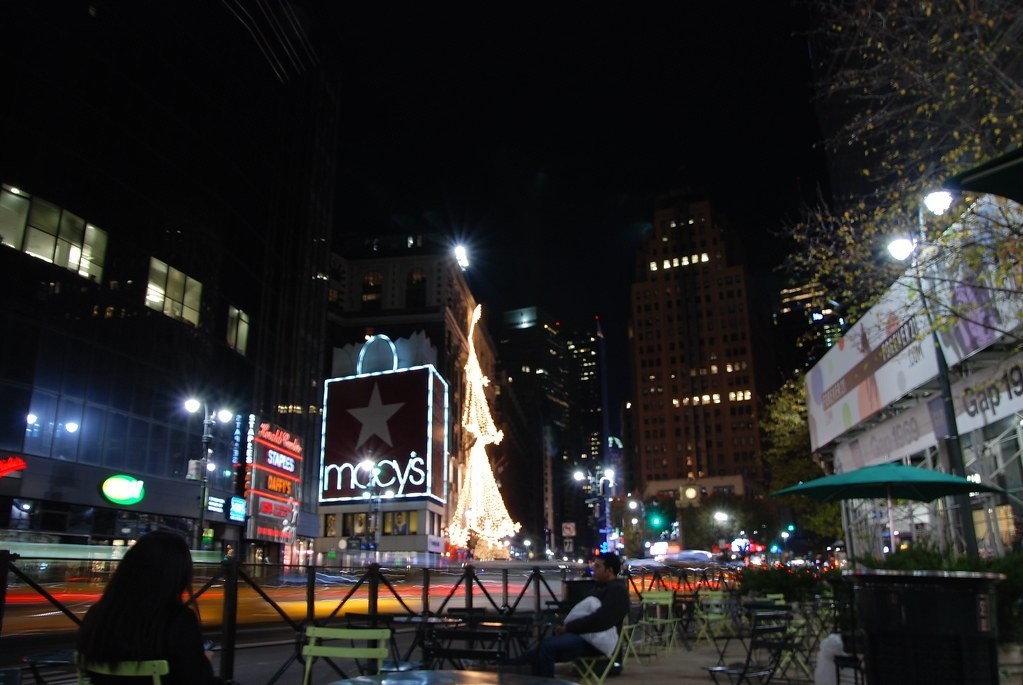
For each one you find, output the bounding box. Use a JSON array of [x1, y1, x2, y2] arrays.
[[787, 518, 795, 536], [651, 517, 662, 527], [771, 541, 779, 552]]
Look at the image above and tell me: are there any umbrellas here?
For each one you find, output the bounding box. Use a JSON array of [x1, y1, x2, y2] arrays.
[[769, 462, 1008, 555]]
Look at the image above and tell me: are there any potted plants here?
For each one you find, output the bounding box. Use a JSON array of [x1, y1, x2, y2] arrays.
[[846, 542, 1011, 684]]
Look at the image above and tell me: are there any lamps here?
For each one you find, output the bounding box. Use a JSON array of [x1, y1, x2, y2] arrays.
[[887, 237, 955, 261], [919, 189, 988, 218]]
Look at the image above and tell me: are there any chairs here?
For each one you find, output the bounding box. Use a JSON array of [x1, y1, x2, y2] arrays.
[[71, 574, 857, 684]]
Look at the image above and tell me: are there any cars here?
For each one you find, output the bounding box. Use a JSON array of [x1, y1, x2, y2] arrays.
[[655, 549, 713, 566]]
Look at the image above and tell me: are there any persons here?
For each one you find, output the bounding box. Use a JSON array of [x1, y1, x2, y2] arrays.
[[394, 513, 408, 535], [73, 530, 215, 685], [535, 551, 623, 679]]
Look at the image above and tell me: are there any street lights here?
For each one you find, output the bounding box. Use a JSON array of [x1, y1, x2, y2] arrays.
[[184, 396, 233, 550], [883, 183, 983, 573]]
